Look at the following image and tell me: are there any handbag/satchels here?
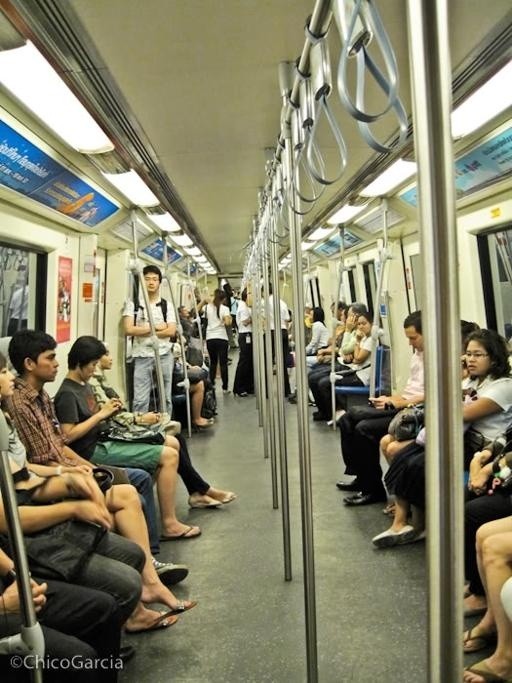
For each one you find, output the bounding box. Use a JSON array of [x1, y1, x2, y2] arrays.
[[24, 517, 105, 584], [107, 410, 170, 445], [485, 426, 512, 493], [350, 405, 398, 420], [417, 389, 477, 446], [388, 405, 424, 441], [191, 318, 207, 338], [336, 370, 353, 376], [180, 365, 207, 382]]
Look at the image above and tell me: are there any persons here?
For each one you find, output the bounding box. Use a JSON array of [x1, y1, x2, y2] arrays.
[[119, 265, 296, 432], [54, 334, 200, 541], [2, 329, 189, 584], [6, 264, 29, 336], [0, 351, 197, 634], [295, 264, 512, 683], [0, 546, 119, 682], [87, 339, 235, 508], [0, 486, 149, 661]]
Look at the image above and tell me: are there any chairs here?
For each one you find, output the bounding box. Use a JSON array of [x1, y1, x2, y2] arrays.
[[146, 392, 189, 409], [331, 344, 385, 406]]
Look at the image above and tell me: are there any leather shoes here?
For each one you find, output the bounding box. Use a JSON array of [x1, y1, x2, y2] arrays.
[[372, 523, 425, 545], [337, 479, 378, 505], [120, 646, 135, 661], [463, 585, 487, 617]]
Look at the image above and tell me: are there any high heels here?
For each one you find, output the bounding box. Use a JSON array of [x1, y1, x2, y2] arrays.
[[193, 416, 213, 433], [233, 392, 247, 397]]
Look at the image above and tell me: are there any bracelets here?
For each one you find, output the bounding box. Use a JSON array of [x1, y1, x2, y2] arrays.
[[55, 463, 61, 475], [4, 567, 20, 583], [141, 415, 144, 424]]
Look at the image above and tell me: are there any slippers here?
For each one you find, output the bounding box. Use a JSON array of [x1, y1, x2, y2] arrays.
[[126, 488, 236, 634], [462, 628, 512, 683]]
